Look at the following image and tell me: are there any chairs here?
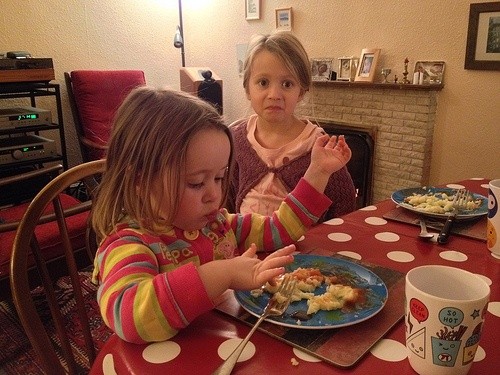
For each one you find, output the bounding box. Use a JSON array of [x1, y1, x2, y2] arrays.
[[67, 69, 146, 157], [0, 162, 109, 375]]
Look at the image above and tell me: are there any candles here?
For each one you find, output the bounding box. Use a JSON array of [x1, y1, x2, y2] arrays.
[[413, 72, 418, 84], [419, 72, 423, 85]]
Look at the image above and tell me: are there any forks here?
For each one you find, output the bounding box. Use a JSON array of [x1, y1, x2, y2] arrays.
[[437, 188, 469, 244], [209, 275, 297, 375]]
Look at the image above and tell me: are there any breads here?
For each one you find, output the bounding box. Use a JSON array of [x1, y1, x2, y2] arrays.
[[404, 194, 479, 214], [265, 267, 363, 314]]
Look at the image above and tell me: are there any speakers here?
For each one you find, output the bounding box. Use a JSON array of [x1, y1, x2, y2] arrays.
[[180, 67, 223, 116]]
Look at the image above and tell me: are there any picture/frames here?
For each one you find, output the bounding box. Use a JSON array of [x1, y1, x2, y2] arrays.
[[310, 59, 333, 81], [245, 0, 260, 20], [275, 7, 292, 29], [355, 49, 380, 82], [337, 56, 354, 80], [464, 2, 500, 70]]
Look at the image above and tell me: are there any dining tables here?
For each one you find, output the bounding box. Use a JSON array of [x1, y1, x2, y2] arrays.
[[117, 179, 500, 375]]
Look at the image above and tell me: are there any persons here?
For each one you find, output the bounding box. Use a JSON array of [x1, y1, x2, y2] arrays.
[[317, 57, 375, 77], [91, 89, 352, 344], [217, 29, 354, 230]]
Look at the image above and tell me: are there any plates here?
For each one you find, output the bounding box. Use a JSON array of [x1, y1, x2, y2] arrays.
[[232, 253, 390, 329], [390, 188, 488, 220]]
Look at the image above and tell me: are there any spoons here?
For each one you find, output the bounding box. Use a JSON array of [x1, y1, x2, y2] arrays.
[[418, 215, 434, 240]]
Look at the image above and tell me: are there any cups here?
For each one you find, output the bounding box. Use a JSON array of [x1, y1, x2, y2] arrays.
[[405, 265, 491, 375], [413, 72, 423, 85], [486, 178, 500, 256]]
